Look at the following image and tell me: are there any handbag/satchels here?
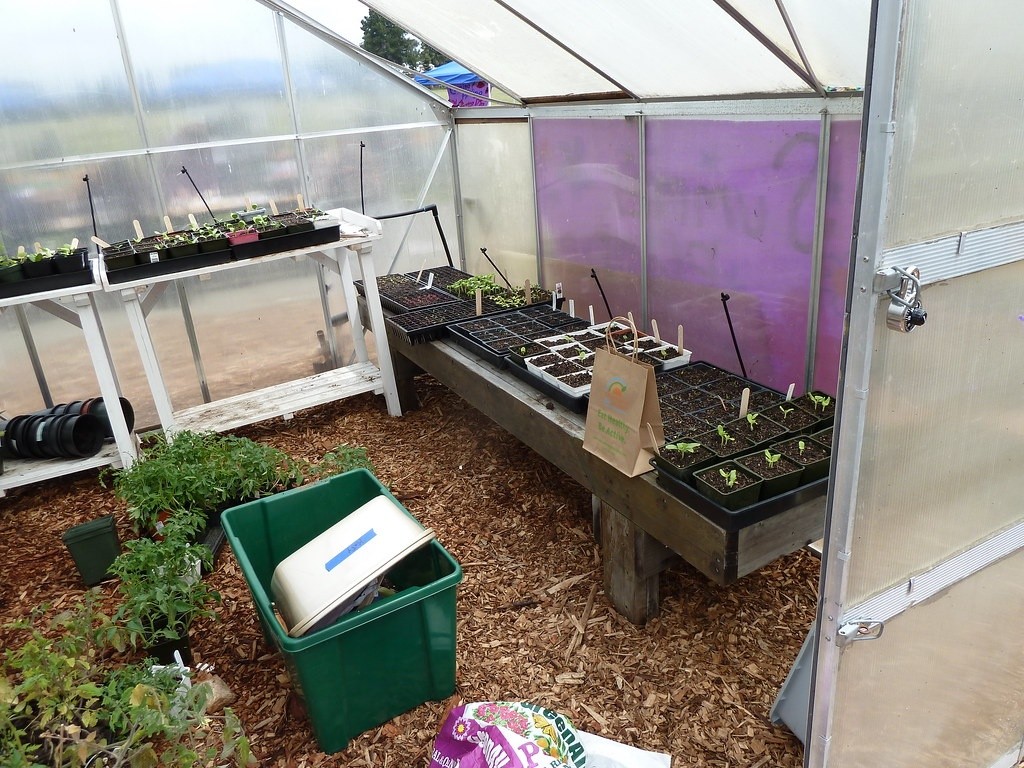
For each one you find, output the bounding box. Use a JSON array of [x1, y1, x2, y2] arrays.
[[582, 316, 664, 477]]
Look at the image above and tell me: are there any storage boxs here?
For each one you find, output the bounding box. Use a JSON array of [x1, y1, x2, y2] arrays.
[[271, 495, 435, 637], [220, 468, 464, 754], [60, 515, 124, 586]]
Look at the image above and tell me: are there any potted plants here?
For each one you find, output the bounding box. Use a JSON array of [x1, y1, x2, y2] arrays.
[[0, 423, 376, 768], [0, 203, 339, 283], [353, 264, 837, 512]]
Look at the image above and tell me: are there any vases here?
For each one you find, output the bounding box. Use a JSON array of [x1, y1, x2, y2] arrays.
[[0, 395, 136, 459]]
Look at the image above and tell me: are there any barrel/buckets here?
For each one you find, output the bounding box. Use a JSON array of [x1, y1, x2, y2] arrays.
[[62, 514, 121, 589], [0, 397, 135, 459]]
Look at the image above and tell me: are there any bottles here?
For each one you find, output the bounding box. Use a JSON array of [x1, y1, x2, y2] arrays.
[[312, 330, 333, 374]]
